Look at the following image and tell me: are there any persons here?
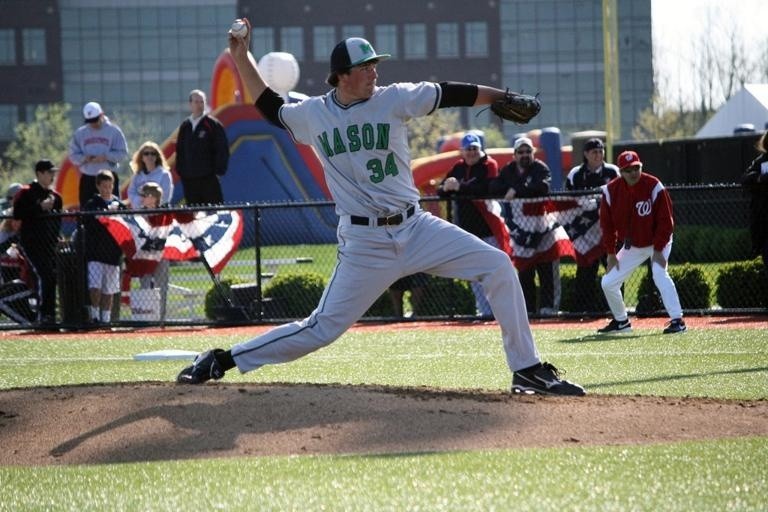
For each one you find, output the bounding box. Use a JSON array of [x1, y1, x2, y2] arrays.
[[560, 138, 623, 321], [176, 17, 587, 398], [489, 136, 562, 315], [1, 102, 173, 334], [176, 89, 229, 207], [436, 133, 509, 322], [596, 151, 687, 336], [743, 130, 768, 270]]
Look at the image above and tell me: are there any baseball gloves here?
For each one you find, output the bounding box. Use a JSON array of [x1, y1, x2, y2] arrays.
[[490, 92, 542, 124]]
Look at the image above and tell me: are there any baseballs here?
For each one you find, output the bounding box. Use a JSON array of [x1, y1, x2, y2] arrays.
[[232, 22, 247, 39]]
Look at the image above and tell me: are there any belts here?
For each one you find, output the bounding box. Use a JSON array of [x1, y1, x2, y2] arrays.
[[347, 202, 421, 228]]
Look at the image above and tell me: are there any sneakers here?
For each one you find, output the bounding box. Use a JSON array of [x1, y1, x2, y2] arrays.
[[90, 304, 112, 324], [510, 361, 590, 397], [176, 347, 226, 384], [597, 317, 632, 334], [662, 318, 687, 335]]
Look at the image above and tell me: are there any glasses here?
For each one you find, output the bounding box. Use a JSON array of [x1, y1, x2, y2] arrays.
[[142, 151, 157, 157]]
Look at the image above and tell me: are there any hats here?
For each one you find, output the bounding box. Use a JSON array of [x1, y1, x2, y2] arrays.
[[34, 159, 61, 174], [616, 149, 644, 171], [461, 133, 482, 151], [513, 137, 534, 152], [82, 100, 104, 124], [582, 137, 606, 163], [329, 36, 393, 72], [5, 181, 32, 198], [136, 181, 164, 200]]
